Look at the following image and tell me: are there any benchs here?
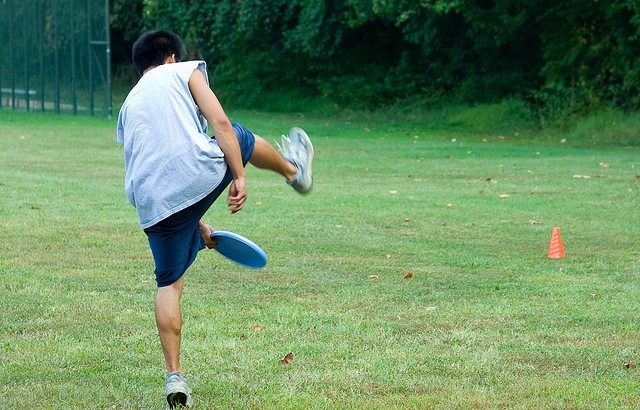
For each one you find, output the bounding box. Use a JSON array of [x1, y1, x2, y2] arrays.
[[0, 88, 36, 108]]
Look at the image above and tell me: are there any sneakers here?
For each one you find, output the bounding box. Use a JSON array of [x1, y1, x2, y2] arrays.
[[272, 126, 314, 194], [164, 371, 193, 410]]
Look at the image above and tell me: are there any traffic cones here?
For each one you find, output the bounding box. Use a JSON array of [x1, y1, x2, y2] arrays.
[[547, 227, 565, 259]]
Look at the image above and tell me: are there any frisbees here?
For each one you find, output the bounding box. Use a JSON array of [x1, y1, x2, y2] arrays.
[[211, 231, 268, 270]]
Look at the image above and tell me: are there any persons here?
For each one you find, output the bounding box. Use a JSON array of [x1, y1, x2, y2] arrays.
[[117, 29, 314, 409]]
